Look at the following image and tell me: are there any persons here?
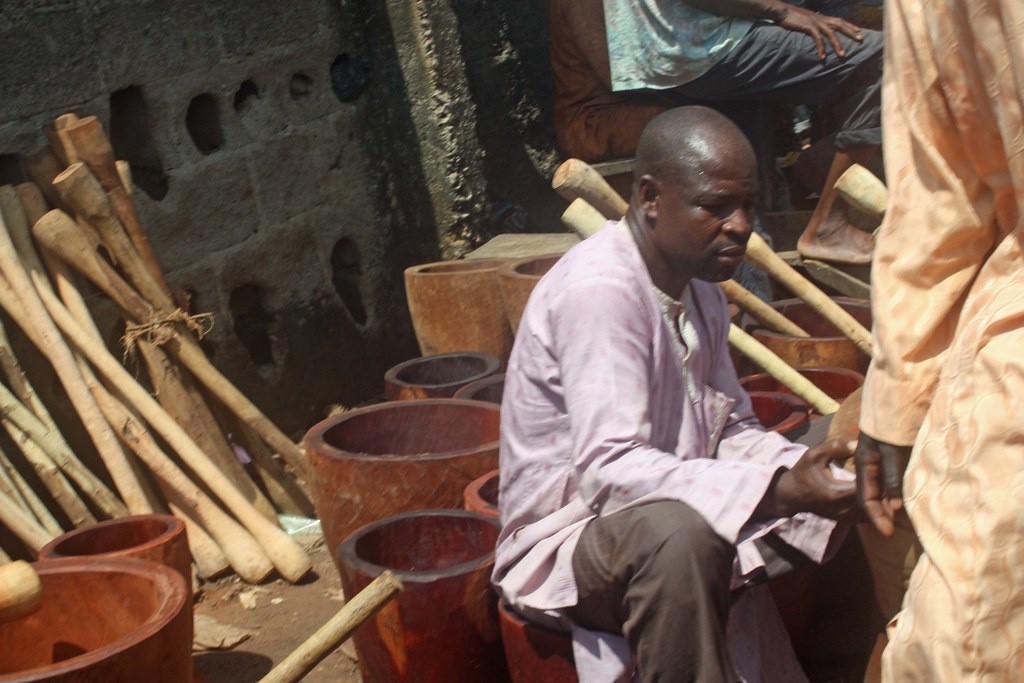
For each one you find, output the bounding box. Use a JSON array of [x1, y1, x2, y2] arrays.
[[486, 103, 879, 683], [599, 0, 889, 266], [853, 0, 1024, 683]]
